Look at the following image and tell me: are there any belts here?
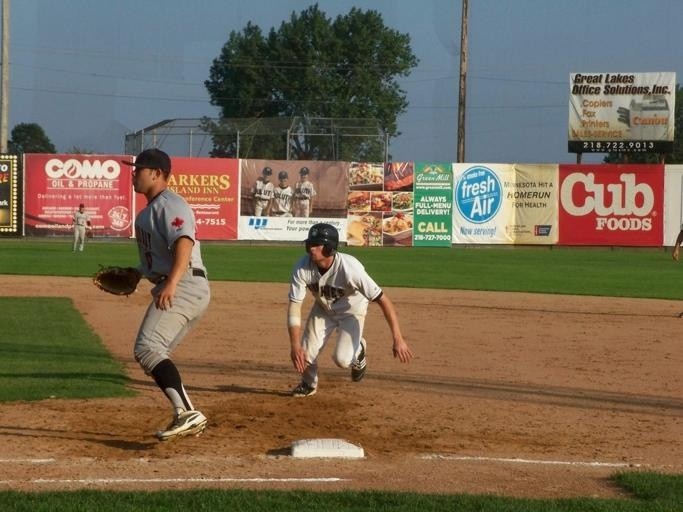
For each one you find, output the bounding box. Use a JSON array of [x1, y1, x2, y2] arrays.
[[299, 198, 310, 199], [273, 213, 285, 216], [260, 199, 268, 201], [158, 269, 206, 282]]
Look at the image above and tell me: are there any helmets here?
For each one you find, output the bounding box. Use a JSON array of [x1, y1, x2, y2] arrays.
[[302, 223, 338, 256]]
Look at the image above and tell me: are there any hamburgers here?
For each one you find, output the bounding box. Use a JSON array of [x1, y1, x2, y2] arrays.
[[348, 192, 367, 212]]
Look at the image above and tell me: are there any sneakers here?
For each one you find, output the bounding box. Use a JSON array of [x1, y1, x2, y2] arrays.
[[157, 410, 208, 441], [352, 339, 368, 382], [291, 384, 314, 397]]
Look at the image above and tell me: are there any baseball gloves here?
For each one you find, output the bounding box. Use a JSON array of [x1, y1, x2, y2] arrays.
[[94, 267, 140, 295], [87, 230, 93, 236]]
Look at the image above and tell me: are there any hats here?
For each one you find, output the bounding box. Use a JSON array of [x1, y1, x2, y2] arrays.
[[279, 172, 287, 180], [263, 167, 272, 175], [121, 150, 171, 171], [300, 168, 309, 175]]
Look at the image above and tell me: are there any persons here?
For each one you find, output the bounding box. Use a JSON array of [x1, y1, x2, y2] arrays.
[[287, 224, 414, 399], [249, 168, 274, 215], [268, 171, 294, 217], [93, 148, 212, 441], [292, 165, 316, 218], [69, 203, 91, 254], [670, 229, 682, 318]]
